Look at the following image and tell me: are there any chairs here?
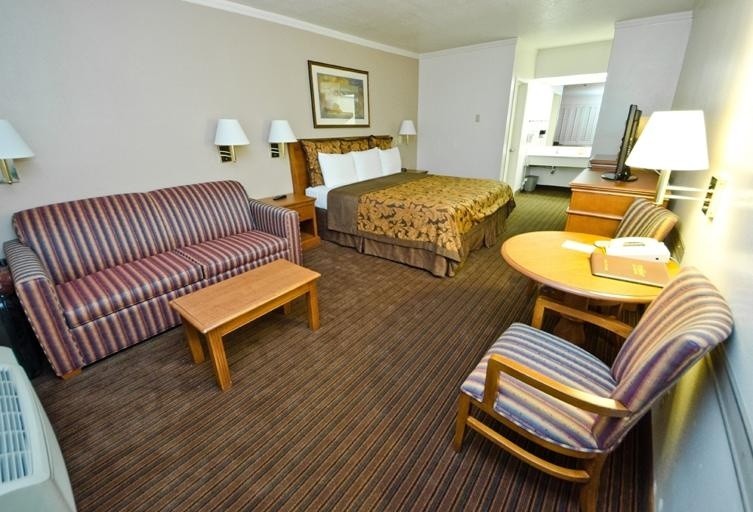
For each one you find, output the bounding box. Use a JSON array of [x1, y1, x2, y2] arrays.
[[525, 198, 678, 324], [452, 265, 733, 511]]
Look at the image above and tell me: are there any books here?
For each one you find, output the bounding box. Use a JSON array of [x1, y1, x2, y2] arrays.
[[590, 253, 671, 289]]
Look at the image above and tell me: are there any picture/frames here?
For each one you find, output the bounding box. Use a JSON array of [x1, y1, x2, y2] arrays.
[[307, 60, 369, 128]]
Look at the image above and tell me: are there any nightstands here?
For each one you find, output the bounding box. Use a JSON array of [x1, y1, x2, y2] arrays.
[[257, 193, 321, 251], [401, 168, 429, 175]]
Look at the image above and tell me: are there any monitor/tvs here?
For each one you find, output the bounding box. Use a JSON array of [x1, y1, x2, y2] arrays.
[[601, 104, 642, 182]]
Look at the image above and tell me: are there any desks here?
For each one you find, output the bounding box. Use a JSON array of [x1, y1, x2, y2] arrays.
[[498, 229, 683, 343]]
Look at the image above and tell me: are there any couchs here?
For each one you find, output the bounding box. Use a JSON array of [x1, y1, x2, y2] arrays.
[[2, 180, 304, 381]]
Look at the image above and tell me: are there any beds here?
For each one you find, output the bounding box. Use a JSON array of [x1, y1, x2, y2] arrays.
[[286, 135, 515, 278]]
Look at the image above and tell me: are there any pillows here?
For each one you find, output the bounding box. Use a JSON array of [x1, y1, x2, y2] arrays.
[[377, 147, 401, 177], [351, 147, 382, 181], [316, 150, 357, 189]]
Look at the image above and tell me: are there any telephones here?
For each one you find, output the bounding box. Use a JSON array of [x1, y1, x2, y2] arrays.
[[594, 237, 671, 262]]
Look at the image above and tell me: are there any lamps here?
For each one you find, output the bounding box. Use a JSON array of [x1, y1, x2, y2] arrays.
[[399, 120, 416, 144], [214, 119, 249, 163], [267, 119, 297, 158], [625, 108, 727, 221], [0, 117, 35, 186]]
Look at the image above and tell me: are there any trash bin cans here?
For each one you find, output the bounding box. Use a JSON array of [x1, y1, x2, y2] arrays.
[[525, 175, 539, 192]]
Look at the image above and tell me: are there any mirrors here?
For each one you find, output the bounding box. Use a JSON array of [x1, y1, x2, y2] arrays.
[[545, 82, 604, 146]]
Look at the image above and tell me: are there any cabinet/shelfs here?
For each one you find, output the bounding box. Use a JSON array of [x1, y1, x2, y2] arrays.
[[564, 168, 673, 239], [587, 154, 618, 168]]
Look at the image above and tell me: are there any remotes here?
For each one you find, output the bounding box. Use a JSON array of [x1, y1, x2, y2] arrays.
[[273, 194, 287, 200]]
